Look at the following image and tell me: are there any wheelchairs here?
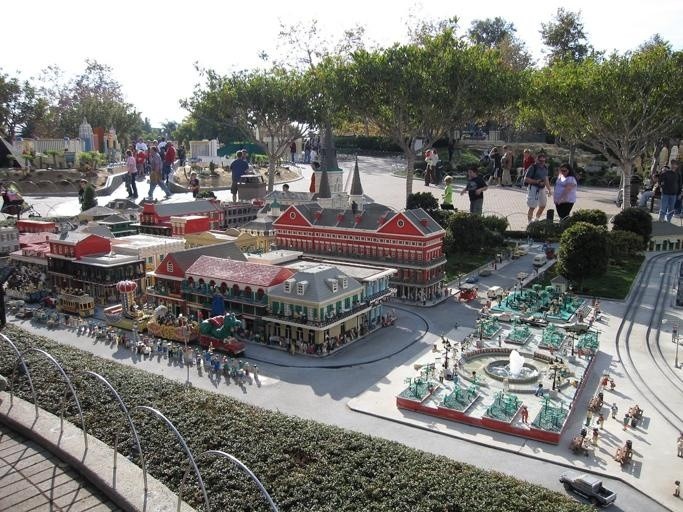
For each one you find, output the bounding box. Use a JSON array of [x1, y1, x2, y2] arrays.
[[616, 182, 639, 207]]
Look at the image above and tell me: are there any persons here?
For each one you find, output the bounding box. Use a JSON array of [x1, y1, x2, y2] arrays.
[[309, 161, 320, 192], [553, 163, 577, 220], [496, 233, 558, 263], [572, 373, 640, 464], [440, 175, 452, 204], [424, 150, 433, 170], [672, 327, 677, 342], [460, 167, 487, 215], [228, 151, 248, 201], [124, 138, 185, 203], [241, 149, 250, 163], [486, 147, 501, 187], [674, 433, 683, 496], [523, 154, 551, 224], [418, 287, 600, 422], [289, 142, 296, 164], [500, 145, 513, 190], [282, 184, 289, 192], [77, 179, 88, 209], [84, 288, 257, 385], [185, 173, 200, 198], [631, 160, 683, 224], [523, 149, 536, 191], [261, 299, 397, 356], [423, 150, 440, 186], [8, 268, 85, 326], [302, 141, 312, 164]]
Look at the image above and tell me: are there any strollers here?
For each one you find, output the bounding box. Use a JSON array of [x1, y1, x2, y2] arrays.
[[0, 181, 42, 219]]
